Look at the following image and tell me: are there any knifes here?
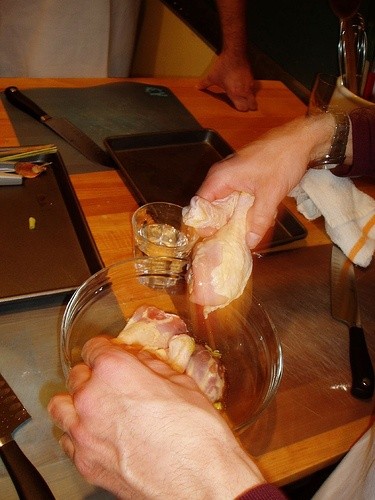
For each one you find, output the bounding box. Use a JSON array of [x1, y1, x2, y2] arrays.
[[331, 242, 375, 400], [0, 373, 56, 500], [5, 86, 116, 168]]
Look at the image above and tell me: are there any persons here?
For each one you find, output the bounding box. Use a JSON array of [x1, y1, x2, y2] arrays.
[[0, 0, 260, 112], [47, 108, 375, 500]]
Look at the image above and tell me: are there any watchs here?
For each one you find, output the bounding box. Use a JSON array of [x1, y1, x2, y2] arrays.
[[307, 107, 349, 171]]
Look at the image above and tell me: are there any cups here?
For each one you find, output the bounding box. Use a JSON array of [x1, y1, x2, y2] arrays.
[[306, 72, 338, 116], [328, 74, 375, 113], [132, 202, 199, 288]]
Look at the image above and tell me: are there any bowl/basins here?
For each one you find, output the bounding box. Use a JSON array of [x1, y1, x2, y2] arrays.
[[59, 256, 283, 437]]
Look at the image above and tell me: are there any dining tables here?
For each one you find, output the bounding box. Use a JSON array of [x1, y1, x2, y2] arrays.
[[0, 72, 375, 500]]
